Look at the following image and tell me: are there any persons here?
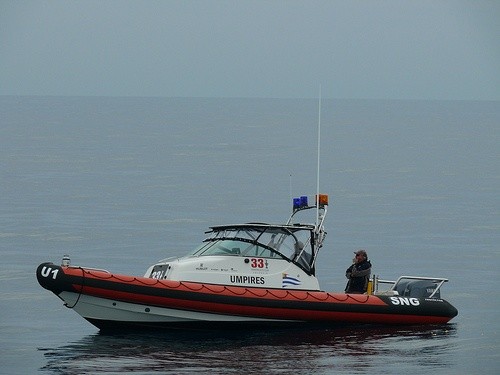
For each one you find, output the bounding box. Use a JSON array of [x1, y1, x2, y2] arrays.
[[290, 240, 315, 276], [344, 249, 373, 294]]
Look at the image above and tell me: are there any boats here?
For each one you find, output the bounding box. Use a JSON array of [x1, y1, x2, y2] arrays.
[[36, 191, 459, 331]]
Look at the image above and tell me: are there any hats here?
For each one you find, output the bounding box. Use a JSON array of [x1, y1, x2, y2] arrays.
[[354, 250, 368, 257], [294, 241, 305, 250]]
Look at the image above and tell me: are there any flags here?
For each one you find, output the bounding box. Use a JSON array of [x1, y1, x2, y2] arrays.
[[316, 194, 329, 206], [292, 196, 308, 209]]
